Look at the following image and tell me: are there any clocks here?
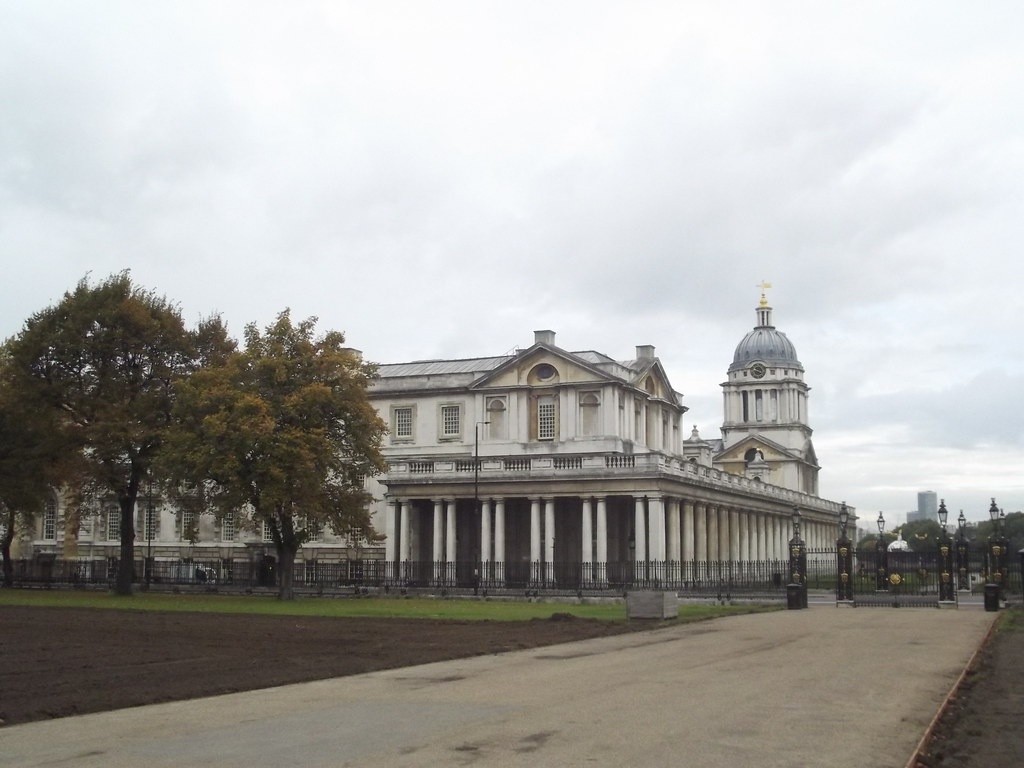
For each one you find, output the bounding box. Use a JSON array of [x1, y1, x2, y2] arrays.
[[750, 363, 766, 379]]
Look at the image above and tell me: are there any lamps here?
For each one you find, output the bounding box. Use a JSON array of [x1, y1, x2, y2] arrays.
[[839, 501, 849, 528], [958, 510, 966, 531], [937, 500, 948, 525], [999, 508, 1006, 528], [791, 506, 802, 526], [628, 528, 635, 549], [989, 499, 999, 526], [877, 511, 884, 534]]
